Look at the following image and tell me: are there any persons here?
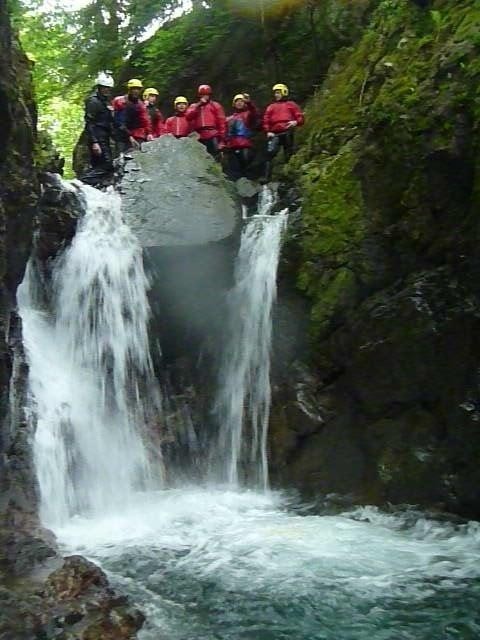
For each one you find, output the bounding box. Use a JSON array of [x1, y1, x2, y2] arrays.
[[108, 79, 154, 157], [262, 83, 304, 178], [141, 87, 162, 143], [184, 85, 225, 164], [224, 92, 262, 180], [71, 72, 113, 171], [162, 96, 192, 139]]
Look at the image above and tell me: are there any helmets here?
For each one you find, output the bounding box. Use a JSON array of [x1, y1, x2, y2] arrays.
[[128, 78, 144, 90], [232, 93, 248, 107], [173, 95, 189, 110], [272, 83, 289, 97], [95, 69, 115, 88], [142, 88, 160, 100], [197, 84, 213, 96]]
